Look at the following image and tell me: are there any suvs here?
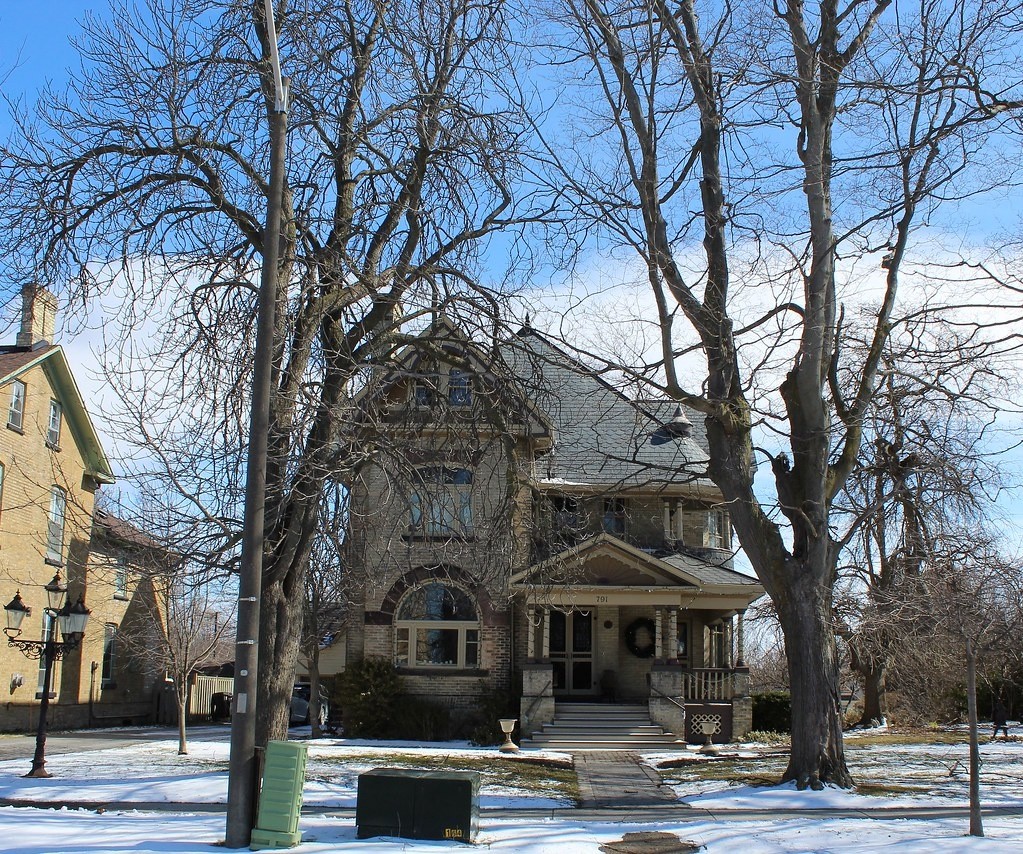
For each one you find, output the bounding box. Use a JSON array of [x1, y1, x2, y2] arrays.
[[289, 681, 330, 726]]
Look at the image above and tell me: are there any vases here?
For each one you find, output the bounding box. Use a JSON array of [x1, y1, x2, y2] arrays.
[[699, 721, 719, 756], [499, 718, 519, 754]]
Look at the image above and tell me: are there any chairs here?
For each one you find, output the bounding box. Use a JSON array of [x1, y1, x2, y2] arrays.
[[598, 670, 619, 703]]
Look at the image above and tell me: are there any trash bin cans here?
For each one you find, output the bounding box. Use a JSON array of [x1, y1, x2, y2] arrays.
[[249, 740, 308, 850], [211, 692, 232, 723]]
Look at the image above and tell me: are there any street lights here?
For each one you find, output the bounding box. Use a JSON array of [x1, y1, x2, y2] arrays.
[[1, 568, 93, 779]]
